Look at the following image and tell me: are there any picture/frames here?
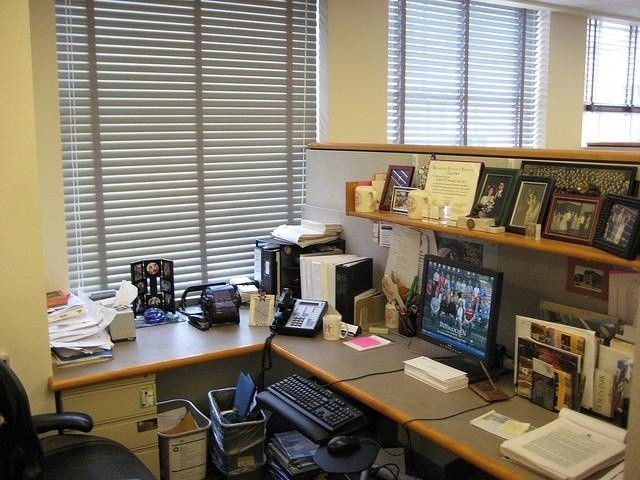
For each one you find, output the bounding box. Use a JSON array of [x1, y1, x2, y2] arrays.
[[592, 192, 640, 260], [521, 161, 637, 196], [566, 255, 610, 300], [542, 192, 604, 246], [379, 165, 415, 211], [249, 294, 274, 326], [390, 185, 418, 216], [505, 174, 557, 235], [472, 167, 521, 226]]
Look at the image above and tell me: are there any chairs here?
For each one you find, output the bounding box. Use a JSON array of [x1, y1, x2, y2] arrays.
[[0, 359, 157, 480]]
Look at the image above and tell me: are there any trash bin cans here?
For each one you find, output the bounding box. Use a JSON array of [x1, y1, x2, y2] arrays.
[[209, 387, 267, 480]]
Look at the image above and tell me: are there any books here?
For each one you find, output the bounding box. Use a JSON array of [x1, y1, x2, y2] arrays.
[[265, 430, 320, 480], [515, 299, 637, 419]]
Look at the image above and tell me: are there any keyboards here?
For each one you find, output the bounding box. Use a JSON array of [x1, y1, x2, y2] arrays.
[[267, 374, 365, 431]]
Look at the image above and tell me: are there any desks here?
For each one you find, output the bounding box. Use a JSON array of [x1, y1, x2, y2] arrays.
[[47, 305, 629, 480]]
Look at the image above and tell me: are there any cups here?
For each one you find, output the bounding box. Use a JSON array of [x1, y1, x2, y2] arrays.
[[354, 186, 382, 212], [321, 313, 349, 343], [407, 187, 433, 219]]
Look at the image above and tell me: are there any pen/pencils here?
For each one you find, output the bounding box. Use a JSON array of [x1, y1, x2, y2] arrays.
[[386, 274, 419, 335]]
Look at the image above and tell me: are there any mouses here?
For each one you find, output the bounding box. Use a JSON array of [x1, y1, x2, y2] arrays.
[[327, 433, 360, 455]]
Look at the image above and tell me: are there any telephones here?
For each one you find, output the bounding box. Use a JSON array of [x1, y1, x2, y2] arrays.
[[270, 288, 328, 337]]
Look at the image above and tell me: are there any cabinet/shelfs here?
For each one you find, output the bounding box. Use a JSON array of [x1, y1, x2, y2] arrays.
[[57, 372, 160, 480]]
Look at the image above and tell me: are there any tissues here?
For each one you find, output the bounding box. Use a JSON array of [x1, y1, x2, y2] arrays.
[[99, 279, 139, 341]]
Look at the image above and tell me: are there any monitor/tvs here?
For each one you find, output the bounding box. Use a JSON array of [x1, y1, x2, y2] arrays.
[[415, 255, 508, 384]]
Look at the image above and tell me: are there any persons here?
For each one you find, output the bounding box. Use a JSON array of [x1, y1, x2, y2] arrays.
[[479, 186, 495, 218], [524, 189, 540, 225], [604, 207, 626, 244], [425, 268, 488, 338], [488, 181, 506, 217], [552, 209, 592, 230]]
